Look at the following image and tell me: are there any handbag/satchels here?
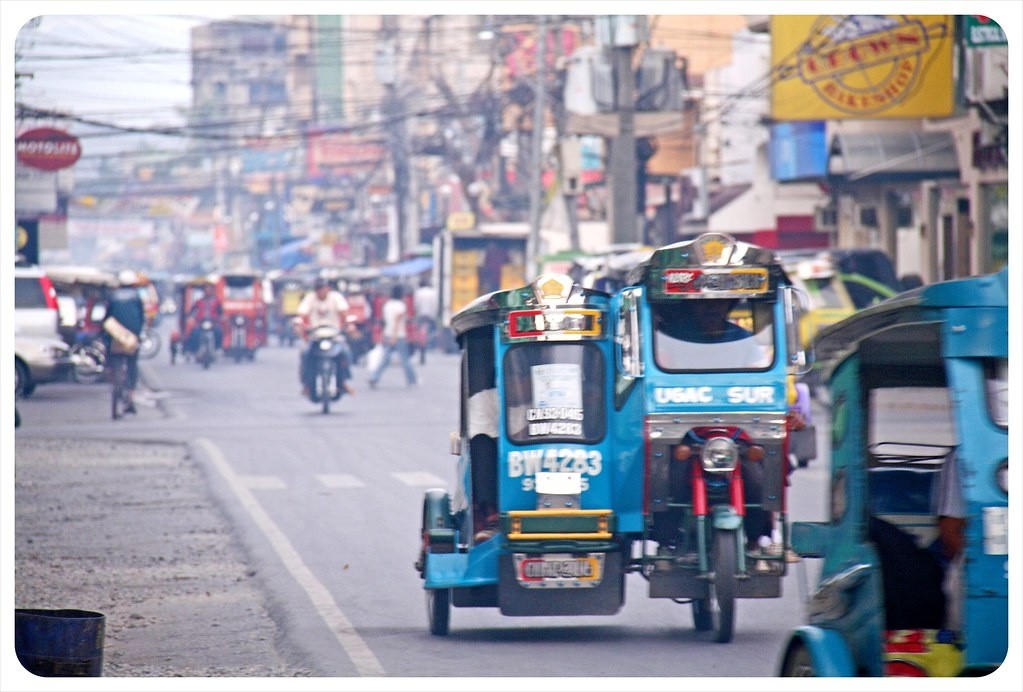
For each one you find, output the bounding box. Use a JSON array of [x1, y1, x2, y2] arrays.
[[365, 341, 385, 371]]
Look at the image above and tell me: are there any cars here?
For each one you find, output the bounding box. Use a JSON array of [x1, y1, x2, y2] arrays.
[[15, 264, 69, 399]]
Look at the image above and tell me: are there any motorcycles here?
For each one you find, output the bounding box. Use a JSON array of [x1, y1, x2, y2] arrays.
[[37, 264, 433, 370], [292, 315, 360, 413], [415, 231, 817, 641], [778, 264, 1008, 678]]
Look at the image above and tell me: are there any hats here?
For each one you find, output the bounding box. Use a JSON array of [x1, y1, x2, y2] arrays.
[[120, 269, 139, 287]]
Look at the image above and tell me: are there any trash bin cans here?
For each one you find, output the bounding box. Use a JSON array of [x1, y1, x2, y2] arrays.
[[16, 608, 106, 677]]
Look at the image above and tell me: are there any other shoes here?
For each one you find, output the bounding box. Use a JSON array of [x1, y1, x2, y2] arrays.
[[122, 393, 134, 407], [105, 367, 114, 382], [337, 382, 354, 394], [302, 384, 312, 396]]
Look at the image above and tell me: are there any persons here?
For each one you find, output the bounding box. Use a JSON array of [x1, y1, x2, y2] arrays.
[[660, 298, 769, 368], [280, 278, 438, 364], [467, 364, 544, 524], [294, 278, 355, 395], [933, 444, 967, 629], [369, 284, 416, 388], [103, 269, 144, 414], [188, 282, 223, 352]]
